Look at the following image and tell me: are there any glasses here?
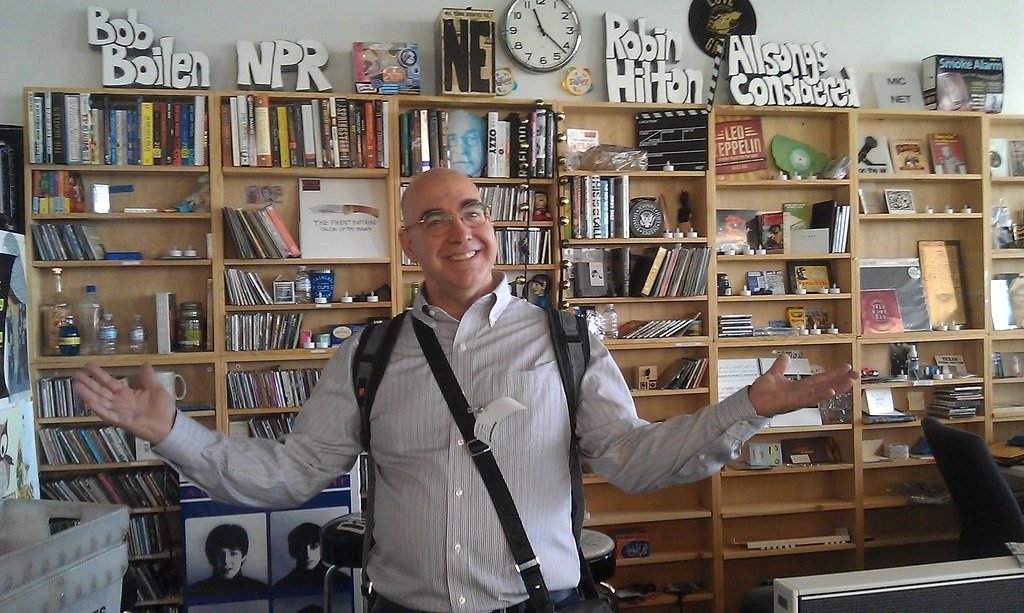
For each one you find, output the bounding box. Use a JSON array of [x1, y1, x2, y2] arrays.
[[403, 202, 492, 237], [449, 134, 485, 147]]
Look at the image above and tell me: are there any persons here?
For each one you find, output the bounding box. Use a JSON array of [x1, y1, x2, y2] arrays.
[[939, 146, 968, 175], [71, 167, 859, 613], [187, 522, 268, 604], [530, 273, 550, 310], [532, 191, 551, 222], [271, 523, 351, 598], [447, 111, 489, 180]]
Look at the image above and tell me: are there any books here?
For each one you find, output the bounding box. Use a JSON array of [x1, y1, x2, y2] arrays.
[[29, 91, 985, 604]]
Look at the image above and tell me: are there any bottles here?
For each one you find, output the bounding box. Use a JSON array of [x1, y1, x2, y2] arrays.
[[76, 285, 104, 356], [127, 315, 147, 354], [175, 302, 207, 352], [907, 344, 919, 380], [98, 314, 120, 354], [57, 315, 80, 356], [407, 282, 419, 310], [39, 268, 73, 355], [295, 266, 311, 304], [601, 304, 619, 340]]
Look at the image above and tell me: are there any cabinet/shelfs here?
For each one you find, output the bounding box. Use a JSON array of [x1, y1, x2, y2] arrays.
[[24, 88, 1024, 613]]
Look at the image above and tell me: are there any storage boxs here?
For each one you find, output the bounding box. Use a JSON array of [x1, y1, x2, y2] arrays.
[[633, 365, 658, 390], [786, 307, 806, 329], [352, 42, 421, 95], [922, 55, 1005, 113], [574, 263, 609, 297], [90, 184, 110, 213], [608, 528, 649, 559], [331, 324, 368, 347], [0, 498, 129, 613], [434, 8, 497, 98], [156, 292, 178, 355], [563, 129, 599, 153], [273, 275, 295, 304], [883, 444, 908, 460]]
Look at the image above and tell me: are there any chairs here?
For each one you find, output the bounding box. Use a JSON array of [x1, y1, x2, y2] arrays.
[[921, 417, 1024, 560]]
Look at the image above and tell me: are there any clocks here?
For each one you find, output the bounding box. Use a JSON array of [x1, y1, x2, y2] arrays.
[[501, 0, 582, 75]]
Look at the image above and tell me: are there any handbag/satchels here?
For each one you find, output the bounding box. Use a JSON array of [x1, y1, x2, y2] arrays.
[[537, 591, 618, 613]]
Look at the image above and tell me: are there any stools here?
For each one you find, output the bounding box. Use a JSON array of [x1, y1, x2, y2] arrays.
[[580, 529, 620, 613], [320, 513, 375, 613]]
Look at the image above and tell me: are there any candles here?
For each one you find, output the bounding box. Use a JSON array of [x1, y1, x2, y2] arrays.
[[314, 290, 379, 303]]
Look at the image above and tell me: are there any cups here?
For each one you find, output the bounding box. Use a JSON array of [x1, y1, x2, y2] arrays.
[[154, 371, 187, 405]]
